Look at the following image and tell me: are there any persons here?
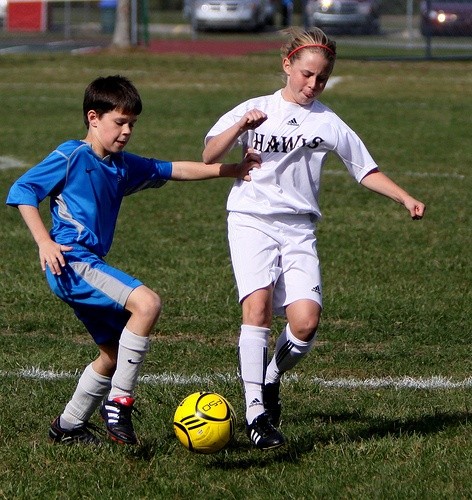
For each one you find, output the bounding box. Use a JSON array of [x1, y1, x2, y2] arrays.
[[6, 76, 262, 445], [202, 32, 425, 445]]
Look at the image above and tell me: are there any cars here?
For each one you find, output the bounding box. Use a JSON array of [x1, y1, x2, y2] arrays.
[[189, 0, 472, 36]]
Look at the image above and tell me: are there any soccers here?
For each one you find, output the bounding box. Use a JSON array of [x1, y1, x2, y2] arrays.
[[174, 391, 237, 452]]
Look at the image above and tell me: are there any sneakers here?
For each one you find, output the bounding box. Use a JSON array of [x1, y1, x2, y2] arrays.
[[49, 417, 107, 447], [245, 412, 284, 449], [99, 394, 137, 445], [263, 363, 282, 424]]
